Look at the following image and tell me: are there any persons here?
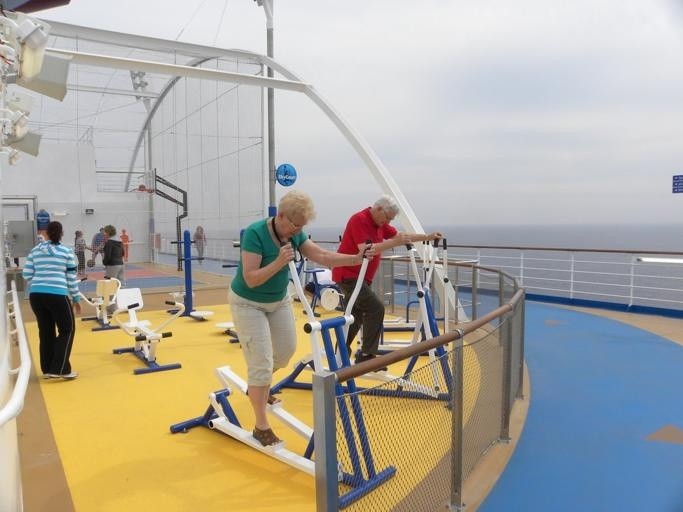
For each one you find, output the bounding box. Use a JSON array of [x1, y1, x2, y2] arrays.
[[193, 225, 208, 266], [74, 229, 93, 282], [119, 229, 134, 263], [228, 189, 376, 453], [21, 221, 82, 380], [91, 225, 126, 290], [331, 194, 445, 373]]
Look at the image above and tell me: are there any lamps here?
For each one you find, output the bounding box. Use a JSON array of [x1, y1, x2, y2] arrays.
[[0, 14, 49, 166]]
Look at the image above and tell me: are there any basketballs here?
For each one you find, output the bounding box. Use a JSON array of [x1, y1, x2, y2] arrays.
[[87, 259, 94, 267], [139, 184, 146, 191]]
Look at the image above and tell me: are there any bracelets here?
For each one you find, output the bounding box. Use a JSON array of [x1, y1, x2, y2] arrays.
[[351, 254, 353, 266]]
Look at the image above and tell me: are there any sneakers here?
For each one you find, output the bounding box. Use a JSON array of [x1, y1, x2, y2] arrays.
[[354, 350, 388, 372], [41, 371, 78, 379], [252, 425, 285, 451], [245, 389, 283, 408]]
